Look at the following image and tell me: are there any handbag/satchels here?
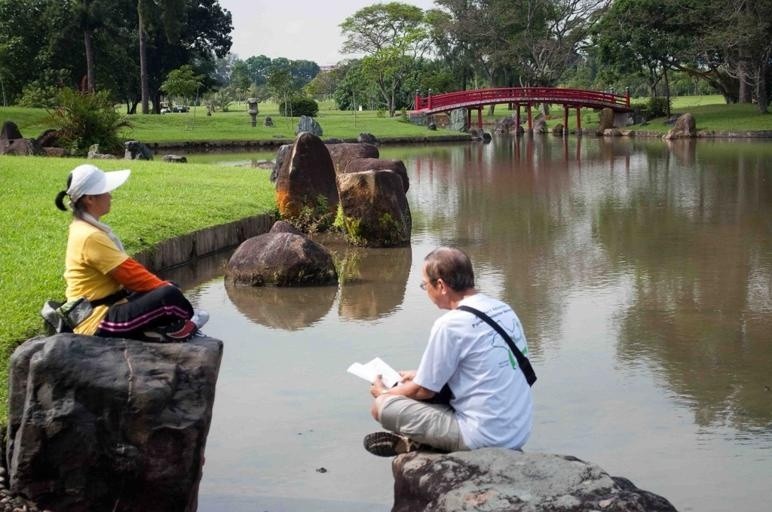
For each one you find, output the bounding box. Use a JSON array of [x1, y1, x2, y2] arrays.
[[424, 384, 453, 409], [39, 300, 88, 334]]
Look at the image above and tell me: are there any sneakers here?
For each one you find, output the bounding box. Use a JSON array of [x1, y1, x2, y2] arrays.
[[364, 431, 423, 457], [192, 312, 209, 328], [145, 329, 164, 343]]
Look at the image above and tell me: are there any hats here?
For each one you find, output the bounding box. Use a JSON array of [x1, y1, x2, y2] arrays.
[[67, 164, 130, 205]]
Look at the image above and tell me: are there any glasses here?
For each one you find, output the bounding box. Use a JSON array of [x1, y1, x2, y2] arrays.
[[420, 280, 436, 290]]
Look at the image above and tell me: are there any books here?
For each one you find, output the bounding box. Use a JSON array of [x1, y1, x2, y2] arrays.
[[347, 356, 405, 390]]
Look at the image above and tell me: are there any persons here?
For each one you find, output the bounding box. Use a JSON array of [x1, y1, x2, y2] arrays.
[[55, 163, 210, 342], [362, 246, 534, 457]]
